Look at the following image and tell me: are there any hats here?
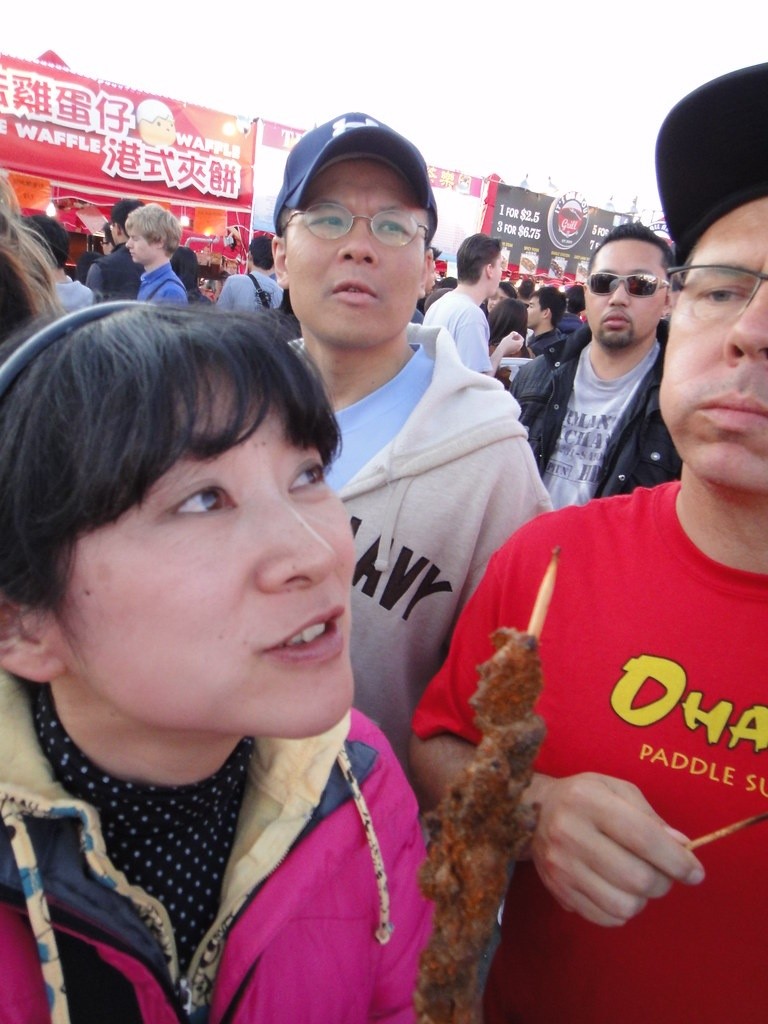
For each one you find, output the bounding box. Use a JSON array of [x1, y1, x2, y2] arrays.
[[655, 64, 768, 267], [273, 112, 438, 248]]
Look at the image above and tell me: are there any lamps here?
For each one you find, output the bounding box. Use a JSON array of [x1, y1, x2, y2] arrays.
[[235, 116, 260, 138]]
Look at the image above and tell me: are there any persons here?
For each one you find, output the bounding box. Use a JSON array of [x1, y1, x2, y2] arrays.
[[88, 199, 211, 304], [1, 165, 93, 336], [431, 236, 585, 378], [410, 64, 768, 1024], [217, 236, 285, 311], [272, 114, 557, 807], [512, 225, 682, 505], [0, 304, 439, 1023]]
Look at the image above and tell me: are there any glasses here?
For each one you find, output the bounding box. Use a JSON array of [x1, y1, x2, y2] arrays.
[[100, 241, 109, 246], [586, 273, 670, 298], [282, 202, 432, 249], [110, 225, 117, 231], [667, 266, 768, 331]]
